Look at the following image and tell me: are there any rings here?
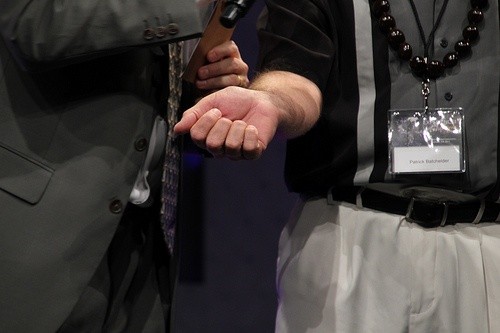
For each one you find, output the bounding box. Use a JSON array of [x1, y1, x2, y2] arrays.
[[237, 75, 244, 85]]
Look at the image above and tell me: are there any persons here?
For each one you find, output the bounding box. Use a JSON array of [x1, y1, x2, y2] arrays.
[[0, 0, 500, 333]]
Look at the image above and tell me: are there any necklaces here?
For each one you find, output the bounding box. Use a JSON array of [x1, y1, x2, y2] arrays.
[[378, 0, 490, 80]]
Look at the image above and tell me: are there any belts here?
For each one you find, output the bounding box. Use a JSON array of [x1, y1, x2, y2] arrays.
[[317, 183, 500, 229]]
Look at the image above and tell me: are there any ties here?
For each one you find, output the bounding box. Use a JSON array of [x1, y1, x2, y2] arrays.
[[158, 40, 185, 258]]
